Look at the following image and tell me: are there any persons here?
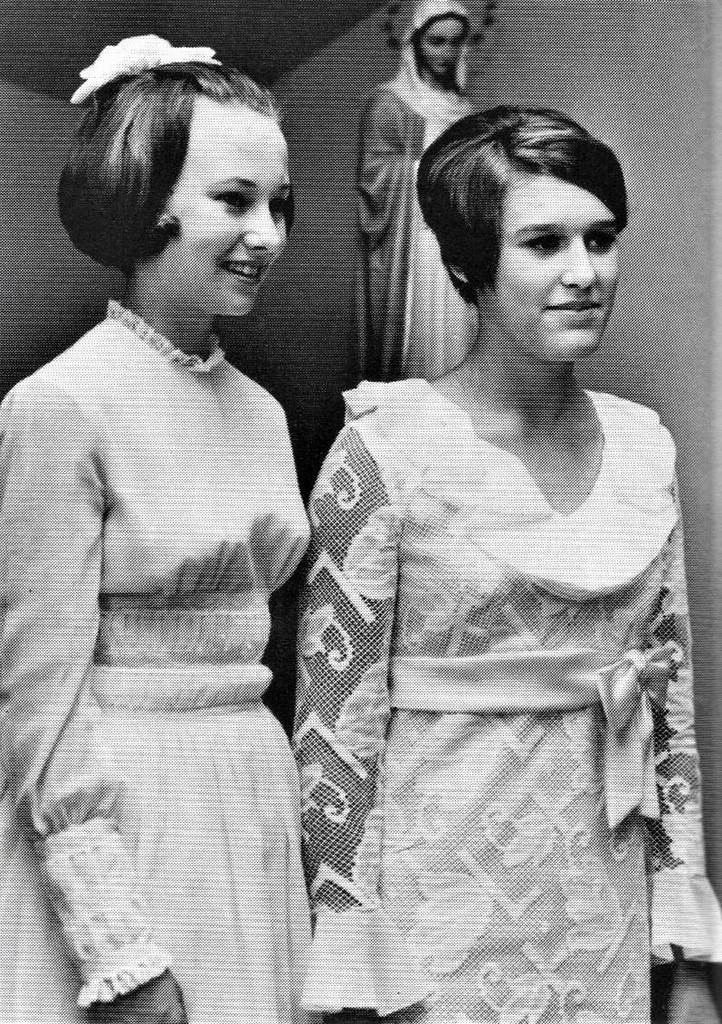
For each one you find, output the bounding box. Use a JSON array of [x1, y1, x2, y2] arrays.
[[301, 104, 722, 1024], [354, 2, 477, 388], [0, 32, 312, 1024]]
